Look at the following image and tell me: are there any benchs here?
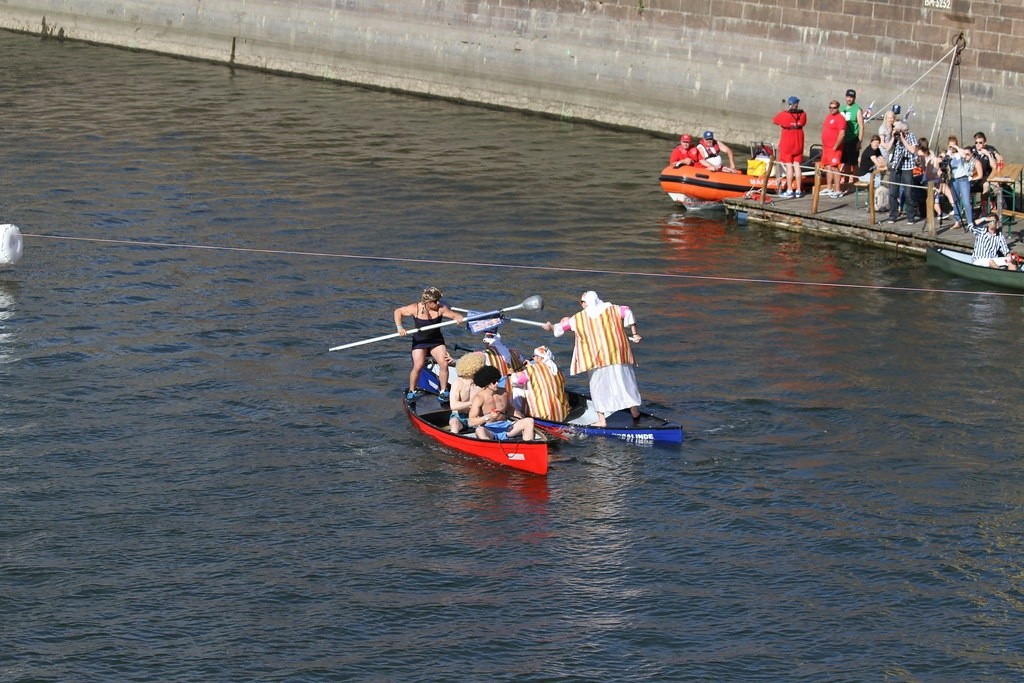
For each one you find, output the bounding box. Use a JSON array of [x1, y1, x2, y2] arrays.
[[853, 181, 870, 210]]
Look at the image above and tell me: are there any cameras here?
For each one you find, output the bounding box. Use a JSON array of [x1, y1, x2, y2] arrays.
[[894, 131, 900, 137]]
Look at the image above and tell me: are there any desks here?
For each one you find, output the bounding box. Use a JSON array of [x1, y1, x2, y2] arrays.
[[986, 162, 1024, 237]]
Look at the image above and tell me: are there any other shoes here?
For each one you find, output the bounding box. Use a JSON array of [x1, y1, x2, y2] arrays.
[[819, 189, 834, 196], [888, 220, 894, 223], [438, 391, 449, 402], [795, 191, 804, 198], [907, 221, 914, 224], [950, 221, 961, 230], [937, 212, 949, 219], [779, 191, 794, 199], [407, 391, 416, 406], [829, 191, 843, 199]]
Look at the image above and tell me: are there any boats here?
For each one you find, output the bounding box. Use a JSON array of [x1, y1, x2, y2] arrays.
[[416, 358, 684, 442], [658, 162, 860, 210], [403, 387, 549, 476], [924, 243, 1024, 291]]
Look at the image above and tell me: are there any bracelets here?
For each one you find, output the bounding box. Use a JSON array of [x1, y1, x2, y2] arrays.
[[396, 325, 404, 330], [484, 415, 489, 421], [631, 332, 638, 336]]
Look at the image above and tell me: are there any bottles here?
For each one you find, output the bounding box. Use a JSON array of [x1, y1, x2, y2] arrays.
[[1000, 158, 1003, 168]]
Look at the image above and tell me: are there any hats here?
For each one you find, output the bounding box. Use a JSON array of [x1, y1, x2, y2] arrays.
[[704, 131, 713, 139], [788, 97, 800, 105], [680, 134, 692, 143], [893, 121, 909, 130], [846, 90, 856, 97]]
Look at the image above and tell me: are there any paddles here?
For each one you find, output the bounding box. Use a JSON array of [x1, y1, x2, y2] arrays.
[[498, 356, 535, 384], [453, 343, 476, 353], [492, 408, 571, 442]]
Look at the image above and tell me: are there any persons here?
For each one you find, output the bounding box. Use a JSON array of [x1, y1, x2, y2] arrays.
[[696, 131, 737, 173], [967, 215, 1017, 273], [392, 286, 642, 441], [774, 88, 1003, 233], [670, 135, 715, 172]]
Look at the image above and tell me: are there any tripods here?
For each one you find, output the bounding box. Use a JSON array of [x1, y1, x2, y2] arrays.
[[922, 172, 966, 233]]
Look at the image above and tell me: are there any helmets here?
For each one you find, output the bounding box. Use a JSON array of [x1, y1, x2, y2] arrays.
[[421, 287, 441, 302]]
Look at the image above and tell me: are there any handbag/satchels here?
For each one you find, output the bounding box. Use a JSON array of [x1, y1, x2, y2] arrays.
[[746, 160, 766, 176]]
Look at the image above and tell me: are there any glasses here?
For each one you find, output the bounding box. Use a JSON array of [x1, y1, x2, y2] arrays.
[[975, 142, 982, 144], [491, 380, 498, 384], [829, 106, 837, 109], [532, 354, 537, 357], [581, 301, 584, 303], [431, 300, 437, 304]]
